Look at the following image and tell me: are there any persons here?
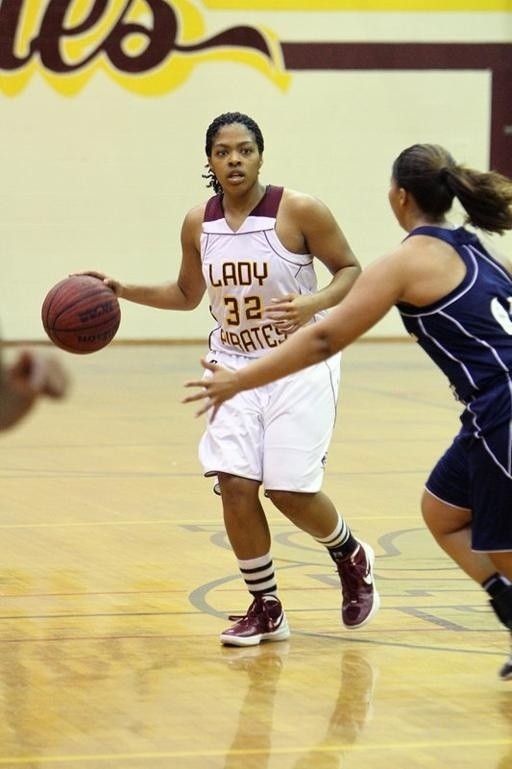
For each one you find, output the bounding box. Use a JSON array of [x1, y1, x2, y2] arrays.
[[70, 113, 380, 647], [0, 341, 69, 432], [182, 144, 512, 680]]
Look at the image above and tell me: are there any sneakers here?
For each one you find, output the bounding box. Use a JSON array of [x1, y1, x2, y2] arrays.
[[219, 594, 291, 648], [335, 537, 381, 630]]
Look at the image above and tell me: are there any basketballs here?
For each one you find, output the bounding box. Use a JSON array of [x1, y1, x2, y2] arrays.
[[42, 275, 120, 354]]
